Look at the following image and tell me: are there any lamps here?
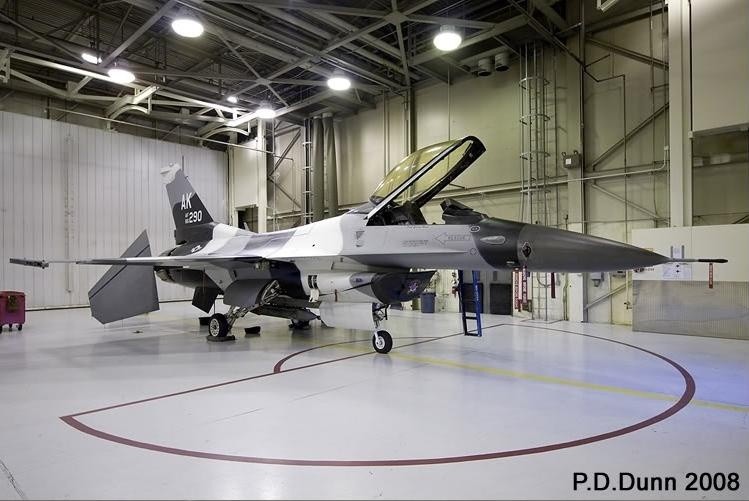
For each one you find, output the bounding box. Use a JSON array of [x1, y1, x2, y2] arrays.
[[171, 5, 204, 38], [433, 25, 462, 52], [327, 67, 351, 92], [108, 58, 135, 84], [256, 99, 275, 120]]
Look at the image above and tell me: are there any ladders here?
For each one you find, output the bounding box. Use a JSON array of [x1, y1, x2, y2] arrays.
[[522, 271, 527, 310]]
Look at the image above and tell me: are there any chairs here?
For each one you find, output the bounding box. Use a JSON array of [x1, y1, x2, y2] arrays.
[[392, 200, 428, 224]]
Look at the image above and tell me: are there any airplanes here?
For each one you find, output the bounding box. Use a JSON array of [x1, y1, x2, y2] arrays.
[[9, 134, 729, 355]]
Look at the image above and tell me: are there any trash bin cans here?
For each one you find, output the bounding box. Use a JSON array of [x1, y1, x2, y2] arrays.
[[528, 301, 532, 312], [421, 293, 435, 313]]
